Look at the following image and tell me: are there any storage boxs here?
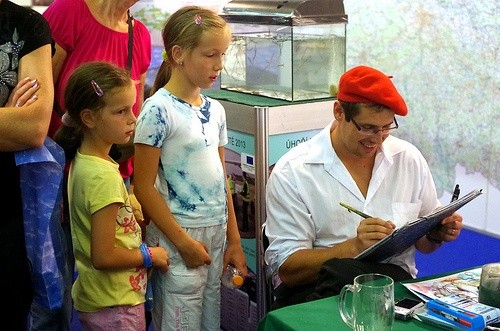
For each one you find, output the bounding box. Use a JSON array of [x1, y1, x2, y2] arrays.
[[217, 0, 349, 103]]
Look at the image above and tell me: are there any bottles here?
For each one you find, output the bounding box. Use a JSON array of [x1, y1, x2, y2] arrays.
[[220, 264, 244, 290]]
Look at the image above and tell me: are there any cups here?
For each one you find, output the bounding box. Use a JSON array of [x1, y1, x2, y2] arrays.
[[477, 263, 500, 331], [337, 273, 394, 331]]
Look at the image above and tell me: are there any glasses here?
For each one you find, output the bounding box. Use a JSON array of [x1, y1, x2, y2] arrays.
[[341, 104, 398, 135]]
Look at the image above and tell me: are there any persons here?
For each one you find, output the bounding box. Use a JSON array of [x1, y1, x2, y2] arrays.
[[51, 63, 169, 331], [0, 0, 56, 331], [39, 0, 152, 224], [134, 7, 247, 331], [265, 66, 463, 313]]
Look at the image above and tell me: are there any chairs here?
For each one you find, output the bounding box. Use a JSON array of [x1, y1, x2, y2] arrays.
[[257, 221, 272, 321]]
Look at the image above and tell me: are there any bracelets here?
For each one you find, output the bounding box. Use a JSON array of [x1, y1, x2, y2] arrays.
[[140, 243, 152, 267]]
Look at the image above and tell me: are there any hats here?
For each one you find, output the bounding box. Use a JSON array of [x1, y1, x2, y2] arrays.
[[337, 66, 407, 117]]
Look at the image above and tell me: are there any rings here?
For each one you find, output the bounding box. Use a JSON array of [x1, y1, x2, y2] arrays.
[[451, 229, 455, 235], [15, 102, 21, 107]]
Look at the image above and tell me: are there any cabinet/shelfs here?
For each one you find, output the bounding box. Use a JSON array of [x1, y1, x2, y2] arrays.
[[201, 87, 339, 331]]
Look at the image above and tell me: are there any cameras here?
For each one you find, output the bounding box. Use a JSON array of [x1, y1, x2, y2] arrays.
[[394, 297, 424, 320]]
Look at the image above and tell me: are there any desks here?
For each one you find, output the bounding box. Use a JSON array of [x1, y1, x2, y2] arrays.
[[255, 264, 483, 331]]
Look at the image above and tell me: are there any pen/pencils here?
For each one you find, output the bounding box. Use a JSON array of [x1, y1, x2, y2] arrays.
[[450, 183, 460, 203], [338, 202, 372, 218]]
